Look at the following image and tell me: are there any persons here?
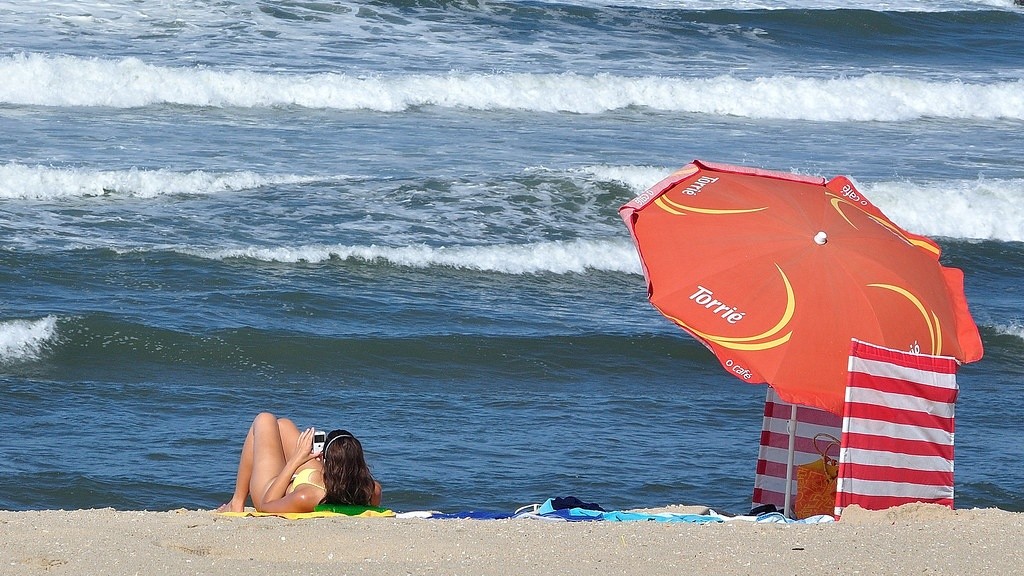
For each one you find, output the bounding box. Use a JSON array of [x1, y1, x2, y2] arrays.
[[215, 411, 382, 513]]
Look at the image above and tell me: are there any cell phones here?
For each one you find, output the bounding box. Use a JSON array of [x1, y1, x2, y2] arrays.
[[313, 432, 326, 455]]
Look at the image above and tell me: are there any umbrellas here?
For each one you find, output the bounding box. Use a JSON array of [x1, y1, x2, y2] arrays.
[[616, 160, 984, 519]]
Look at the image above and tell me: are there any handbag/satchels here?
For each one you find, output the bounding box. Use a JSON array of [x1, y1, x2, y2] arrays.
[[795, 433, 841, 520]]
